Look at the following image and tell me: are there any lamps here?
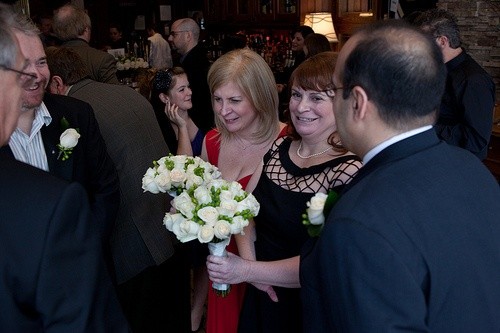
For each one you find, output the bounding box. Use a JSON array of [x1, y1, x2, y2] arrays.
[[303, 12, 339, 44]]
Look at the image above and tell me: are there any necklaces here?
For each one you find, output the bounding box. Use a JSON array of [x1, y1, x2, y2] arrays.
[[296, 141, 341, 159]]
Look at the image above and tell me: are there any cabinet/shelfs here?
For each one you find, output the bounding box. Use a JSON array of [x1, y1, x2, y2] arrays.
[[201, 0, 378, 58]]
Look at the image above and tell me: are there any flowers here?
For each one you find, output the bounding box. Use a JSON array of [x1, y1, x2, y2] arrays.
[[163, 179, 260, 297], [57, 128, 80, 161], [113, 54, 149, 80], [301, 183, 347, 239], [141, 153, 223, 211]]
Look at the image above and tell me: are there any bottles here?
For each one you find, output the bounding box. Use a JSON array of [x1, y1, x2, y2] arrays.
[[207, 27, 226, 62], [246, 33, 295, 73]]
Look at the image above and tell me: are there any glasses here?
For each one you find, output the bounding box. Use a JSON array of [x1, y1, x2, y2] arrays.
[[170, 30, 189, 37], [0, 63, 39, 88], [324, 83, 344, 100]]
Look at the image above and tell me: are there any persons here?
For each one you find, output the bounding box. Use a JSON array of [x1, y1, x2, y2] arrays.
[[168, 18, 211, 136], [416, 10, 495, 161], [44, 4, 121, 86], [36, 14, 62, 47], [0, 22, 131, 333], [299, 25, 500, 333], [0, 2, 122, 287], [199, 47, 291, 257], [303, 33, 331, 57], [148, 66, 213, 157], [108, 23, 129, 49], [276, 26, 314, 92], [45, 45, 193, 333], [146, 24, 172, 71], [206, 50, 365, 333]]
